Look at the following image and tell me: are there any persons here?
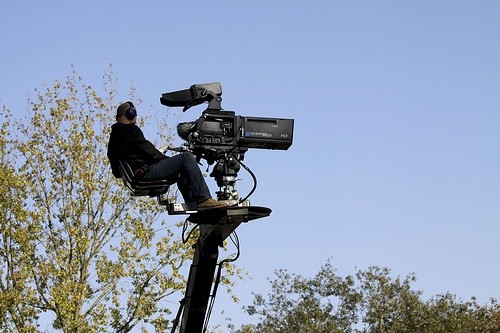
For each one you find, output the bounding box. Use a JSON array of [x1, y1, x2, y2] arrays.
[[107, 101, 229, 209]]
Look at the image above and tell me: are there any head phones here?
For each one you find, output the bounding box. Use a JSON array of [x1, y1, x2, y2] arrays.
[[125, 101, 137, 121]]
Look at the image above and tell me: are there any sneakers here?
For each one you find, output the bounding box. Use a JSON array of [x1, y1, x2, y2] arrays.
[[190, 198, 229, 210]]
